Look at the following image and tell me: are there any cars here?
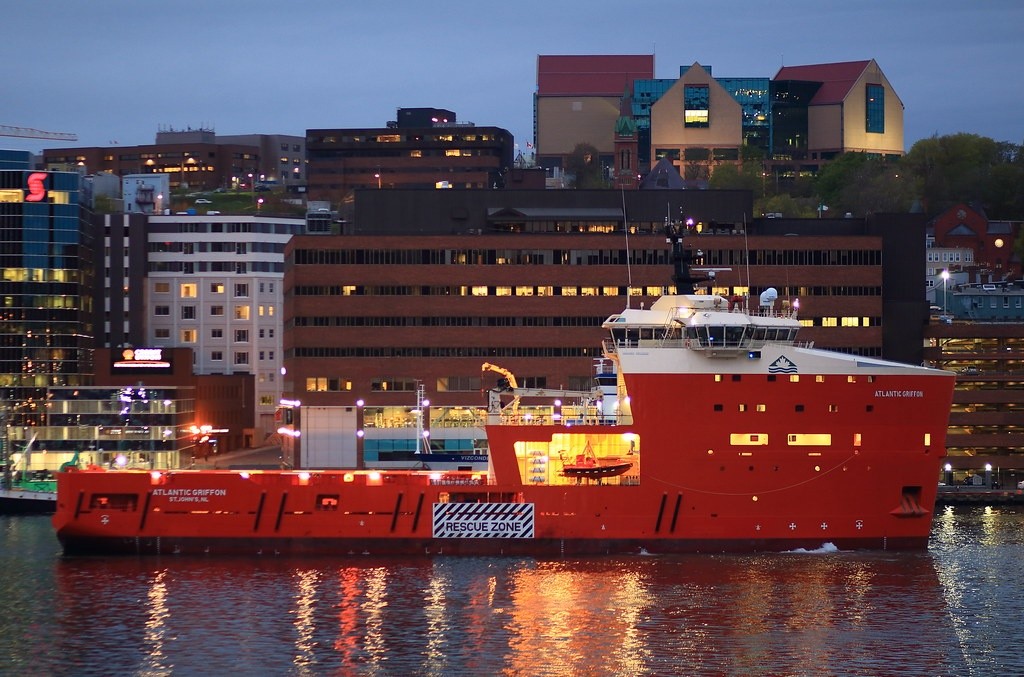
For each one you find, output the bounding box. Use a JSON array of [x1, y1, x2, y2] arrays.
[[1017, 481, 1024, 490]]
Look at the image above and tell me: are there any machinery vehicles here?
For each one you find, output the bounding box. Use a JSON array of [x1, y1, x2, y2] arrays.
[[481, 362, 533, 426]]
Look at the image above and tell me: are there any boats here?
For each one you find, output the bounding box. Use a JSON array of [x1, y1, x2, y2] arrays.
[[1, 410, 57, 516], [51, 178, 961, 559]]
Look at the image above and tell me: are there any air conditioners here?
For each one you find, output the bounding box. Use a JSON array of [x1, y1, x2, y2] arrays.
[[305, 211, 341, 235]]
